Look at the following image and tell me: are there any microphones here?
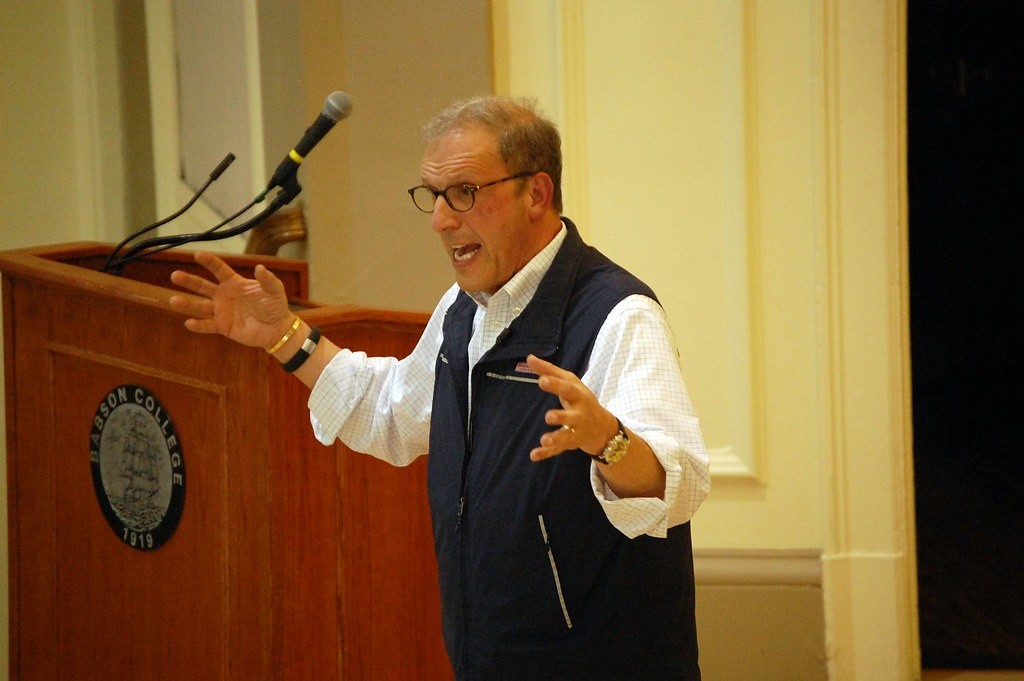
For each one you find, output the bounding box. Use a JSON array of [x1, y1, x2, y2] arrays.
[[98, 152, 237, 273], [267, 91, 354, 191]]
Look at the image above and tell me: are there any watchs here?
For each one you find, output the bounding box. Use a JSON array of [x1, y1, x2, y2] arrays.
[[575, 415, 632, 466]]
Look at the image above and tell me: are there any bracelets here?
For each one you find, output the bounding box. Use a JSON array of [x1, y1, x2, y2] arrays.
[[266, 316, 321, 374]]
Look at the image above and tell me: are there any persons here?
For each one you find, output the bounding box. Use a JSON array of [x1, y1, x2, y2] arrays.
[[169, 94, 712, 681]]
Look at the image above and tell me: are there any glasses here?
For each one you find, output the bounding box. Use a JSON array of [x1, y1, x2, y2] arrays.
[[408, 171, 532, 214]]
